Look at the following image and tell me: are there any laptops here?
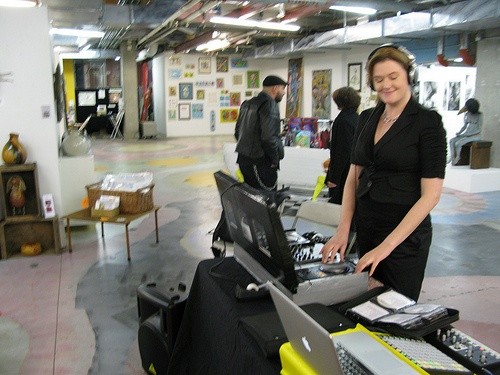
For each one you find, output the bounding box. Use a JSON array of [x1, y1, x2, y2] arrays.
[[266, 281, 422, 375]]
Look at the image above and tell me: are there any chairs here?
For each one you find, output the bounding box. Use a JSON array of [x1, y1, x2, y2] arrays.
[[292, 201, 358, 257]]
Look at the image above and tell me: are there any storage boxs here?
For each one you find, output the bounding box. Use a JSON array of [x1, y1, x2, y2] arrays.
[[0, 163, 42, 219]]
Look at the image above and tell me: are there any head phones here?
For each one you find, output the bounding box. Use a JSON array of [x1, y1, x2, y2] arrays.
[[364, 43, 419, 92]]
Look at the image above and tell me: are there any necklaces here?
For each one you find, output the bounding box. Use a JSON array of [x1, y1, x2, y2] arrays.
[[382, 111, 400, 123]]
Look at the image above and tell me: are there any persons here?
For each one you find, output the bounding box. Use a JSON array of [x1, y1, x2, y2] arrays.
[[235, 76, 287, 193], [319, 43, 446, 304], [447, 98, 483, 165], [324, 87, 361, 205]]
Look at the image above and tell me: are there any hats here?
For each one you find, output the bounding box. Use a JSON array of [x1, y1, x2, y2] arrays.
[[263, 76, 287, 86]]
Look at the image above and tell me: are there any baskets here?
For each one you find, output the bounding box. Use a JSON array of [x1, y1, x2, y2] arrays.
[[86, 181, 154, 213]]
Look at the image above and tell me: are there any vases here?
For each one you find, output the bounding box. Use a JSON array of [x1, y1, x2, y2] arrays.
[[2, 133, 26, 165]]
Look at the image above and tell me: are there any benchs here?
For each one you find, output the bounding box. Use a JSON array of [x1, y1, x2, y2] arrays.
[[454, 140, 492, 169]]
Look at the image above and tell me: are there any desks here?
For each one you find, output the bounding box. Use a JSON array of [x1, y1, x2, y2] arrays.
[[62, 206, 161, 261], [276, 146, 331, 197], [59, 150, 96, 227], [164, 257, 283, 375], [0, 216, 60, 258]]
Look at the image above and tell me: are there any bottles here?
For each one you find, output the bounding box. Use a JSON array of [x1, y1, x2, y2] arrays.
[[2, 133, 27, 165], [62, 130, 88, 156]]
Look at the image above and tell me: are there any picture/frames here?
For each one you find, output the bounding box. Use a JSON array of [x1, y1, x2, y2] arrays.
[[179, 83, 193, 99], [179, 103, 191, 119], [348, 62, 362, 92], [285, 58, 302, 118]]
